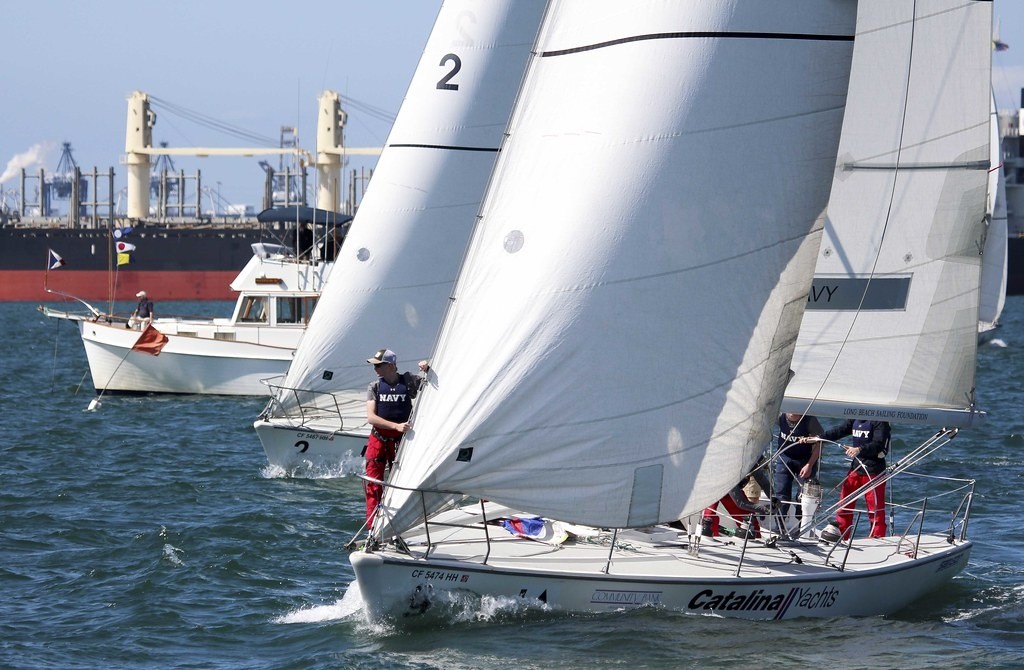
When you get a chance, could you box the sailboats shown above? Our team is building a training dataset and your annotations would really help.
[254,0,549,481]
[342,1,992,630]
[978,89,1008,346]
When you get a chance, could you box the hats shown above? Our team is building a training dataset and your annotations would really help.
[367,349,397,365]
[136,291,146,298]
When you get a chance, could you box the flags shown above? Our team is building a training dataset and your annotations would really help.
[111,227,133,240]
[115,242,136,253]
[49,250,65,271]
[118,254,135,265]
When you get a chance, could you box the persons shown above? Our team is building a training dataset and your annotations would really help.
[824,420,892,538]
[134,290,153,330]
[704,461,781,539]
[320,224,344,261]
[361,348,429,553]
[773,412,825,538]
[293,221,312,260]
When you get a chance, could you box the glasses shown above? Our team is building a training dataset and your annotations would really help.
[374,362,388,368]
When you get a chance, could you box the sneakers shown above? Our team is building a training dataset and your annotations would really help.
[388,536,407,553]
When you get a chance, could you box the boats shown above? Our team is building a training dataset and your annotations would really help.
[37,138,355,397]
[0,90,352,302]
